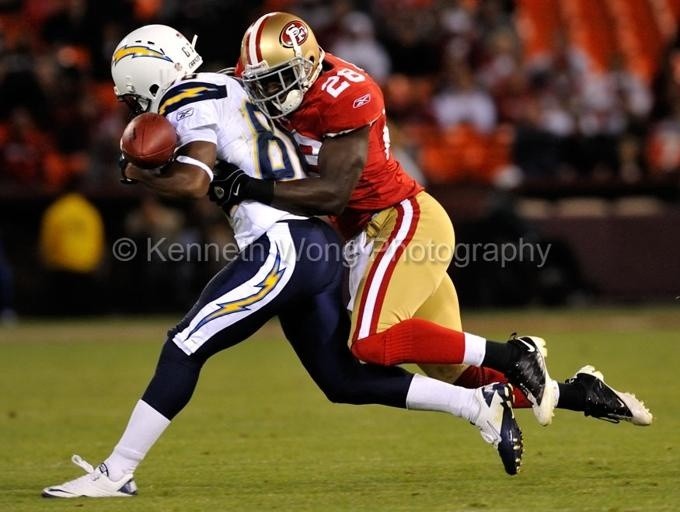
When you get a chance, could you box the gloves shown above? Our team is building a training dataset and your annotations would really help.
[206,158,274,210]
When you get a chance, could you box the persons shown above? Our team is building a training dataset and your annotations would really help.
[203,11,652,426]
[39,22,524,498]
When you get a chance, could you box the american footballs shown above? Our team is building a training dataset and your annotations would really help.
[120,112,177,169]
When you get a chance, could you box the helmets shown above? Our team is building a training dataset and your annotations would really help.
[111,24,203,115]
[242,12,325,119]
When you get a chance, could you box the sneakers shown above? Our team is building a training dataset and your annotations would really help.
[475,382,525,476]
[570,364,653,426]
[42,456,138,498]
[509,336,561,426]
